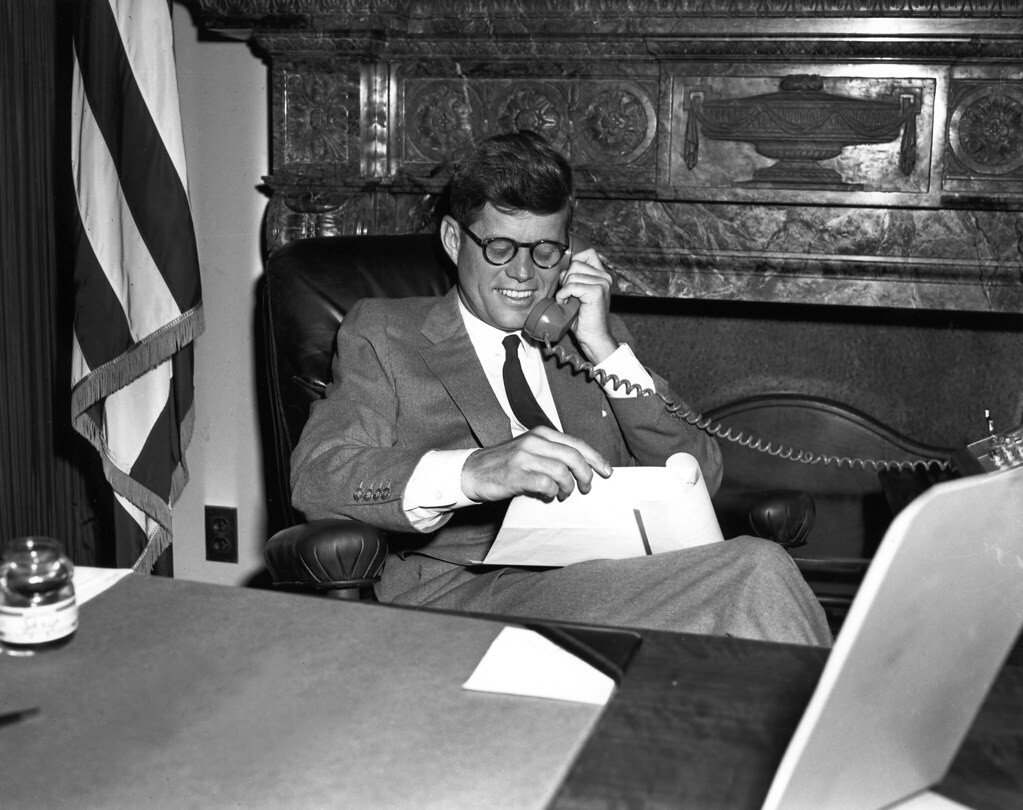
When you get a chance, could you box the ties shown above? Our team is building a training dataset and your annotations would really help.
[502,334,560,432]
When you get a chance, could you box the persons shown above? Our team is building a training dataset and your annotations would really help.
[289,129,834,648]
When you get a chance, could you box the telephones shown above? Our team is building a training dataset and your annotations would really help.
[524,232,590,342]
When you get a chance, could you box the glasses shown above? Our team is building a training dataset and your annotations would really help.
[460,221,570,269]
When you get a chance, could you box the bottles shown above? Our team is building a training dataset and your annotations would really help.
[0,536,79,650]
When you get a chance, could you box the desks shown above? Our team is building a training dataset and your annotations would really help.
[0,567,1023,810]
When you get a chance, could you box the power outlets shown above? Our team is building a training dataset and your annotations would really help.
[205,505,237,563]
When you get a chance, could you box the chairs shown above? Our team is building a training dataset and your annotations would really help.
[263,236,817,603]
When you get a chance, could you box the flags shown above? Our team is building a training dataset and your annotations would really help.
[1,1,205,574]
